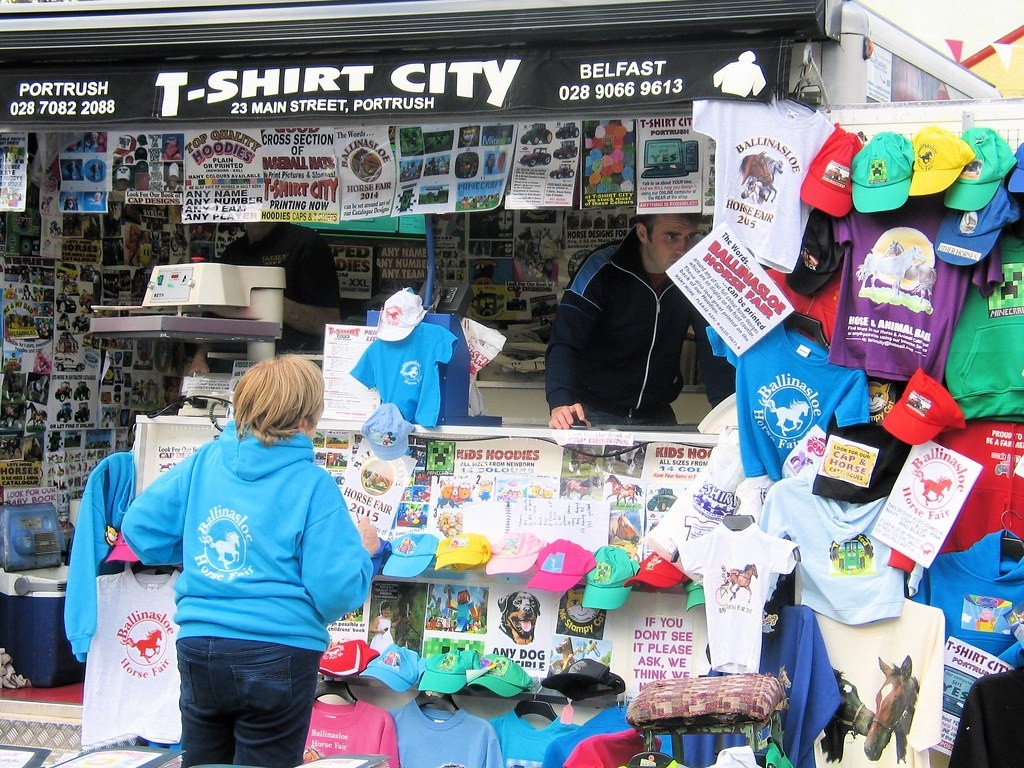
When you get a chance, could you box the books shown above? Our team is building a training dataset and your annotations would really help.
[51,747,187,768]
[0,745,52,768]
[296,753,391,768]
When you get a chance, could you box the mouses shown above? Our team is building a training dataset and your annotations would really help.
[569,417,587,430]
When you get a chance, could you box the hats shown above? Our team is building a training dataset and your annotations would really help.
[786,127,1024,295]
[882,369,967,445]
[376,290,424,341]
[360,403,414,460]
[367,533,704,611]
[320,640,627,702]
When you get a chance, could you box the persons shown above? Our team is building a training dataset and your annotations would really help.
[544,213,736,430]
[122,354,380,768]
[617,216,626,228]
[188,223,340,373]
[450,214,465,241]
[164,163,182,191]
[113,166,134,191]
[473,241,488,255]
[163,137,182,160]
[3,146,11,161]
[539,228,553,256]
[370,601,401,652]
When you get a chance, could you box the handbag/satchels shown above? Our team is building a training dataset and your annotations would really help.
[461,319,506,416]
[627,672,783,733]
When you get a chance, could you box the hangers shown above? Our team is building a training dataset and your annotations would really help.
[415,690,460,712]
[130,559,174,577]
[784,62,816,113]
[782,291,830,354]
[625,728,673,768]
[714,717,785,768]
[670,495,801,563]
[1000,509,1024,563]
[514,680,557,722]
[313,676,357,707]
